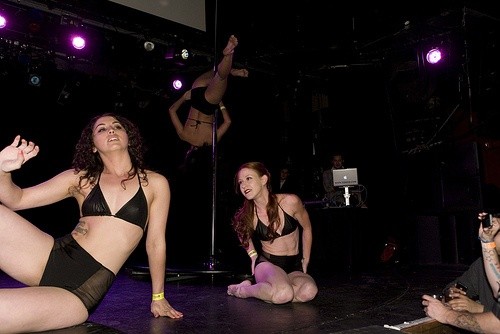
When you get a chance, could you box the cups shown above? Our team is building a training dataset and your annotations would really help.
[449,282,468,299]
[430,294,445,306]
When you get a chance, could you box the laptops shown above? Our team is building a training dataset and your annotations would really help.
[333,168,358,187]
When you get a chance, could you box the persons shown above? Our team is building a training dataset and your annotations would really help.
[226,161,319,304]
[168,35,249,165]
[422,211,500,334]
[274,153,360,202]
[0,112,183,333]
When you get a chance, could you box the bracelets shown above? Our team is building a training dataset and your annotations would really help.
[248,249,257,257]
[481,241,496,248]
[152,291,165,301]
[220,105,226,110]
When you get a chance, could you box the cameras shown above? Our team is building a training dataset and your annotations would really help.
[482,214,492,228]
[455,282,468,292]
[431,295,443,302]
